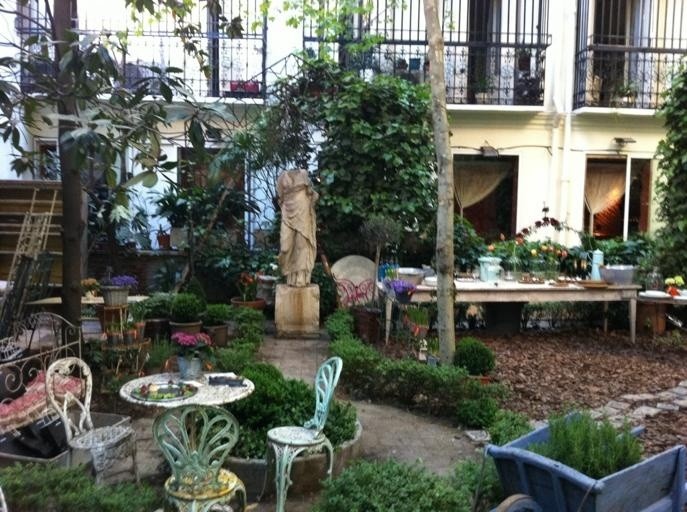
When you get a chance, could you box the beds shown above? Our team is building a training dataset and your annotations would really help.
[0,312,85,446]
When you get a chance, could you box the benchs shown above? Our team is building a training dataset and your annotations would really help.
[225,81,261,98]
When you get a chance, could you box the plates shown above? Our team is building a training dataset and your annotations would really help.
[130,382,199,403]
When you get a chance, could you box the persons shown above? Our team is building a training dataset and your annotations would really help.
[275,167,319,288]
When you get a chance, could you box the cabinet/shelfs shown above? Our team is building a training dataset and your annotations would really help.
[101,306,145,378]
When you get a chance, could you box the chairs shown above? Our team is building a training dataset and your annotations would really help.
[256,356,343,512]
[150,404,248,511]
[320,253,378,312]
[43,357,142,490]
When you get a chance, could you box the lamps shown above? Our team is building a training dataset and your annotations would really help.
[615,134,637,153]
[478,145,499,159]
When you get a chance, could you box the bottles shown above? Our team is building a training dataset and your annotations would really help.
[379,259,399,283]
[591,248,604,283]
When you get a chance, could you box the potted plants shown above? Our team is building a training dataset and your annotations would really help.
[104,290,231,347]
[99,319,145,343]
[214,361,363,500]
[454,336,495,384]
[351,211,403,343]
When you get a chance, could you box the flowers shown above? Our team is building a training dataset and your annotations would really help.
[664,275,685,288]
[99,273,137,289]
[171,330,210,355]
[239,269,257,302]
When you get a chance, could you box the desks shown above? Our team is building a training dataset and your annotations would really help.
[374,277,637,346]
[118,368,256,448]
[637,291,687,335]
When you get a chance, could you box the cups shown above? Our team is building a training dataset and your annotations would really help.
[477,256,502,283]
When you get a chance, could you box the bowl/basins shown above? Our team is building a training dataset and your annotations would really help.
[598,263,640,285]
[397,267,425,286]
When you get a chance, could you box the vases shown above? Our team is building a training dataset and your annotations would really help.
[665,286,680,297]
[103,286,129,305]
[229,297,265,311]
